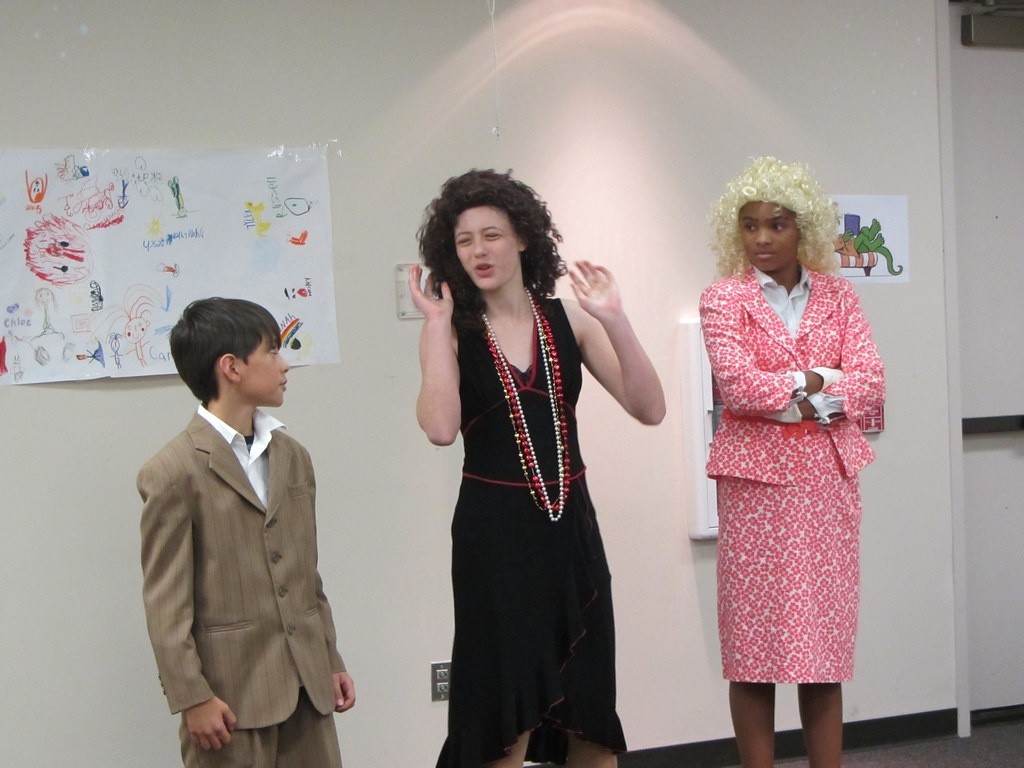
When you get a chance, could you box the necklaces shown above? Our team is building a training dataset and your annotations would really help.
[479,284,573,526]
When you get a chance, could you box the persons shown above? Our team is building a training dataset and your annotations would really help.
[692,152,887,768]
[406,165,667,768]
[139,296,357,768]
